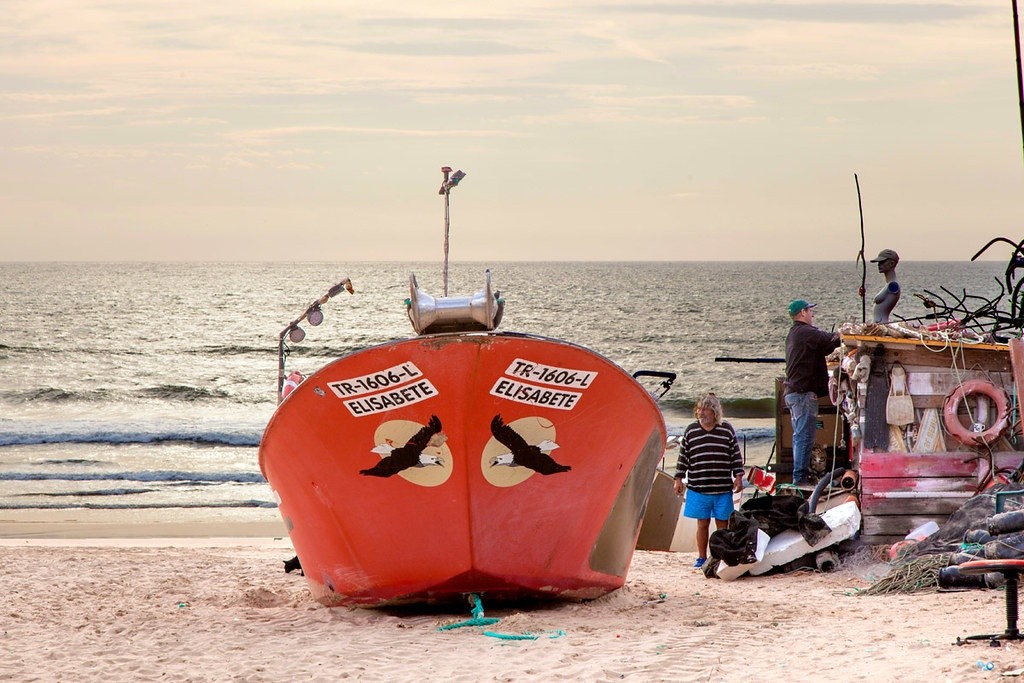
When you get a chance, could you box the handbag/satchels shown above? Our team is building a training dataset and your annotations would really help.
[886,381,914,425]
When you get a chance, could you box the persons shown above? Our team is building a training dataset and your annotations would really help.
[869,249,901,325]
[673,393,745,569]
[782,299,844,486]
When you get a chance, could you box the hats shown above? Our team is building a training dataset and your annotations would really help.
[870,249,900,263]
[788,299,817,316]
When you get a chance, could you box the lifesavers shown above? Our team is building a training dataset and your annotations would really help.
[944,380,1012,447]
[281,370,304,402]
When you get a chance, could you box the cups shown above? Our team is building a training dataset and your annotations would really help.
[973,423,985,432]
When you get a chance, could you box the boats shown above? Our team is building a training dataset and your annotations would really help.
[257,168,678,609]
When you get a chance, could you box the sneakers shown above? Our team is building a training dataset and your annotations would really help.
[694,557,706,570]
